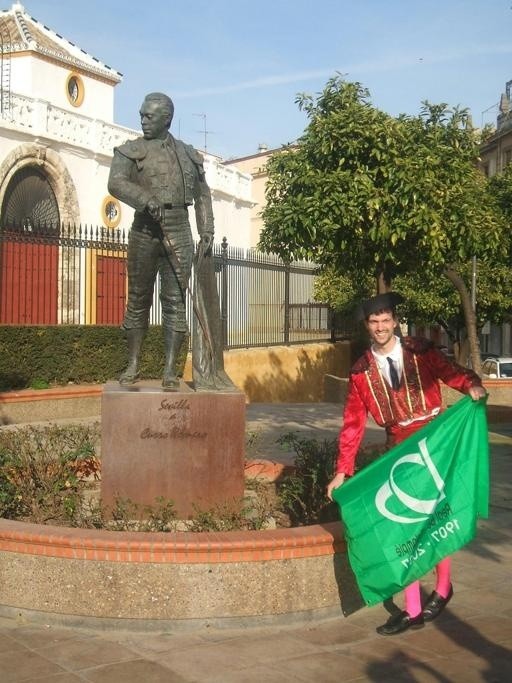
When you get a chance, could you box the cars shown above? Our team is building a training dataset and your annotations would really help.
[482,357,512,380]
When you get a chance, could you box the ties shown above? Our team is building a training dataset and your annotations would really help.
[386,356,401,391]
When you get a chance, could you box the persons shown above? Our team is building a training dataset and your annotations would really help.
[107,92,215,392]
[327,292,487,635]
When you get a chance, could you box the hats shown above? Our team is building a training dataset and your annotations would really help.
[360,291,403,321]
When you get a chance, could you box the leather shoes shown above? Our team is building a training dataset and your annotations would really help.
[421,582,453,622]
[376,610,425,635]
